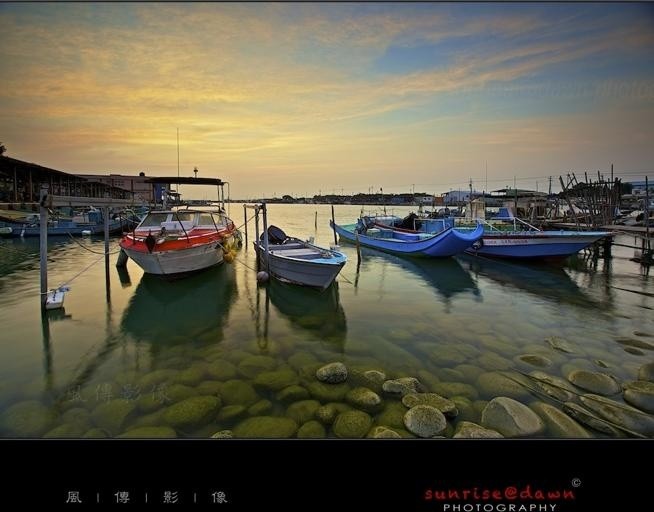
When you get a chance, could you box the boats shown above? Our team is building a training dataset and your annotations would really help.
[0,204,236,273]
[329,215,626,256]
[253,225,348,290]
